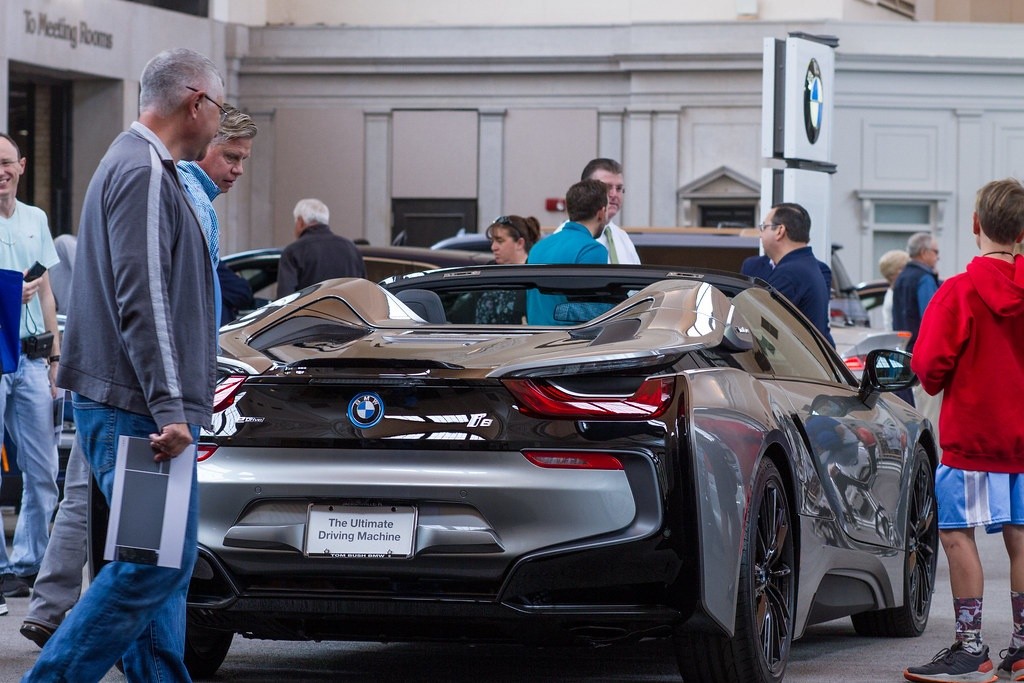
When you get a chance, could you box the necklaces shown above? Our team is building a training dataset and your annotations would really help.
[983,251,1013,256]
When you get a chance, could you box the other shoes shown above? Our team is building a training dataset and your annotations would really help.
[20,621,57,649]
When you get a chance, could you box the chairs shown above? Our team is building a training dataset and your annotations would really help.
[395,289,448,325]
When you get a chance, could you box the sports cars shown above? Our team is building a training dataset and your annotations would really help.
[85,263,942,682]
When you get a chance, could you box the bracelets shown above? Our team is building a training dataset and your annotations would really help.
[48,355,60,363]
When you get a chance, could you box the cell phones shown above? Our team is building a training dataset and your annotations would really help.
[24,261,47,283]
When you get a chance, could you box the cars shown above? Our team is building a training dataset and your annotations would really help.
[220,225,913,412]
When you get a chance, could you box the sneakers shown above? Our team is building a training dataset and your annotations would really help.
[0,573,31,598]
[17,574,37,588]
[903,640,999,683]
[994,646,1024,682]
[0,590,8,615]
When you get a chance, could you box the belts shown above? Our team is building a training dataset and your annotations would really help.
[20,339,28,354]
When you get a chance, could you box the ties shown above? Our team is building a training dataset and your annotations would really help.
[603,226,619,264]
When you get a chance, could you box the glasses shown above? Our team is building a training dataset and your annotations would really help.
[186,85,228,123]
[493,215,523,237]
[925,248,939,255]
[606,183,625,194]
[758,224,781,232]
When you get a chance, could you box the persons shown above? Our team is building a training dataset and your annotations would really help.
[892,233,948,462]
[474,214,541,325]
[524,178,610,325]
[276,199,367,300]
[0,46,257,683]
[555,159,644,297]
[879,250,910,331]
[904,177,1024,683]
[742,254,833,308]
[760,203,828,346]
